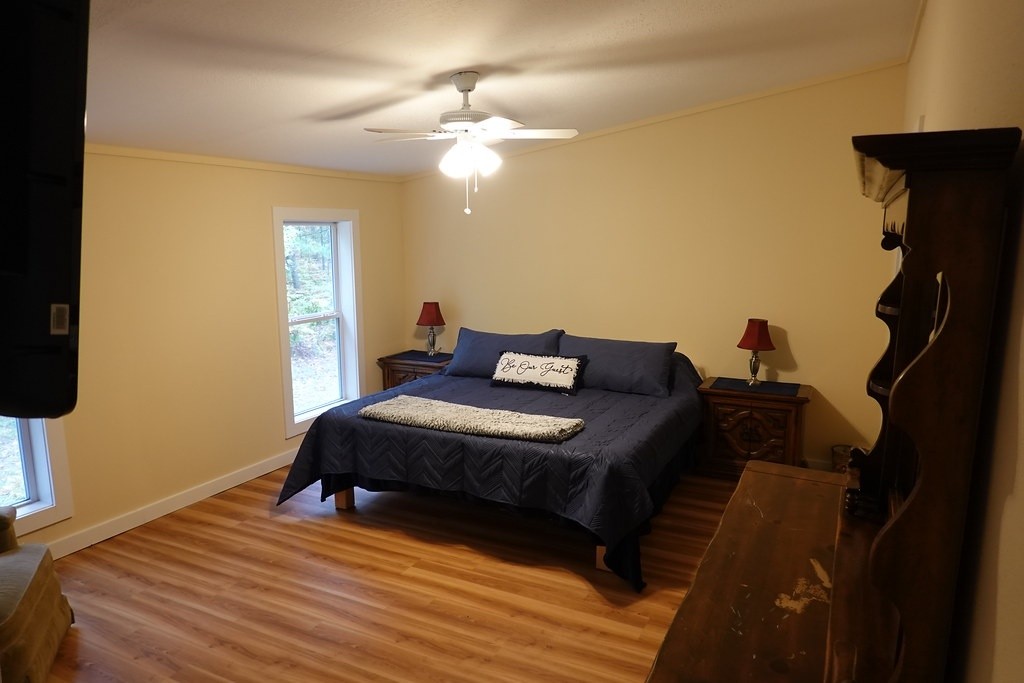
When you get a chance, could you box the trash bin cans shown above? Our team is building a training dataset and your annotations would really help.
[831,444,869,473]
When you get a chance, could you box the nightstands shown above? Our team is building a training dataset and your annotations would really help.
[697,377,812,481]
[377,350,454,391]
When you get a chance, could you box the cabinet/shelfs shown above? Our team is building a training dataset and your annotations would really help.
[642,127,1024,683]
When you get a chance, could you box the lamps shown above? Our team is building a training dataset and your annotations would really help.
[439,134,504,213]
[416,302,446,356]
[736,318,776,387]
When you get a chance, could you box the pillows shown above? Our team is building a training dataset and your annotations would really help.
[558,333,677,397]
[489,351,587,396]
[444,327,567,377]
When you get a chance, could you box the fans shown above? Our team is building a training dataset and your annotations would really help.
[364,71,579,143]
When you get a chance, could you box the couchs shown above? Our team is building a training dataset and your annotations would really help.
[0,506,74,683]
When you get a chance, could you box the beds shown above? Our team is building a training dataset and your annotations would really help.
[275,350,703,592]
